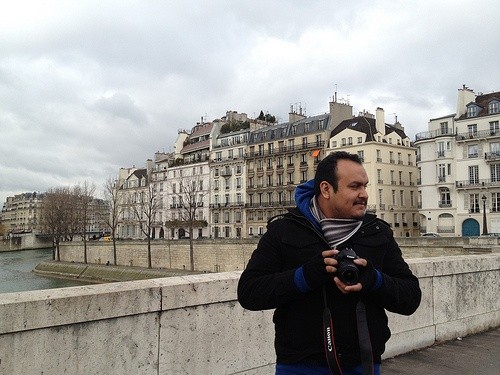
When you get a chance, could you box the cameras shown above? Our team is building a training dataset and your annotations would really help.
[330,247,361,286]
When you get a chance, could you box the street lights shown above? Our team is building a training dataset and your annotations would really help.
[480,194,491,235]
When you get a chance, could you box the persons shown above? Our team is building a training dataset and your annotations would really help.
[237,152,422,375]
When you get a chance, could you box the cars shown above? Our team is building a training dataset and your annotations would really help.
[421,233,440,238]
